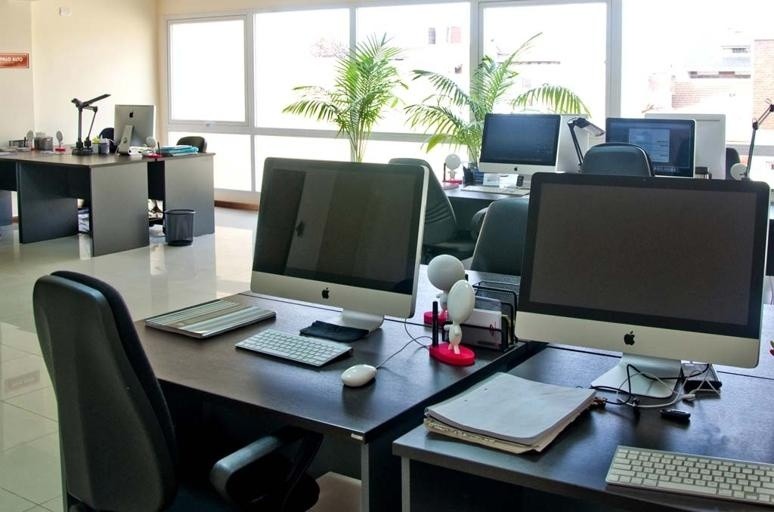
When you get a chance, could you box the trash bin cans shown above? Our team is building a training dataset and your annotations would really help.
[164,209,196,246]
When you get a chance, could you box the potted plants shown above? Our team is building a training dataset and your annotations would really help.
[92,134,101,154]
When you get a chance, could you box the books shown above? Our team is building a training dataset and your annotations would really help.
[424,372,597,455]
[156,145,199,157]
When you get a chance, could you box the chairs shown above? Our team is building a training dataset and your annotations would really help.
[470,197,529,276]
[97,127,116,156]
[578,143,654,178]
[176,136,208,152]
[32,272,320,507]
[388,158,475,262]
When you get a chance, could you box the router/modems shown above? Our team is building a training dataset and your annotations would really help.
[681,363,721,393]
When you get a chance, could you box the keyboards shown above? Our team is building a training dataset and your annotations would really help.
[461,185,531,196]
[606,444,773,507]
[235,328,351,368]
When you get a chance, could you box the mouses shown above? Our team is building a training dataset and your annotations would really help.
[340,363,378,389]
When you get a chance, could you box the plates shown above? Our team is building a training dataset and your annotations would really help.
[129,153,142,156]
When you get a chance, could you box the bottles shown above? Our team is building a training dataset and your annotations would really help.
[694,166,712,179]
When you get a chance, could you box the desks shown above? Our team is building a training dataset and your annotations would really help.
[148,151,217,242]
[439,179,530,225]
[390,346,774,512]
[0,148,156,256]
[134,293,526,509]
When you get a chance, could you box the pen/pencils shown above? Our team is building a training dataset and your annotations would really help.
[432,300,438,347]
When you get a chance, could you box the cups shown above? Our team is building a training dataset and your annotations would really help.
[98,143,110,155]
[128,146,141,154]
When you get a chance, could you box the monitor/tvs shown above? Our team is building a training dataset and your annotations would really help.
[249,156,429,341]
[558,114,589,168]
[604,116,698,176]
[114,104,156,155]
[514,171,770,398]
[476,111,563,188]
[648,113,728,180]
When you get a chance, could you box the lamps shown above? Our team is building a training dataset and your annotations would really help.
[71,94,111,156]
[567,117,605,167]
